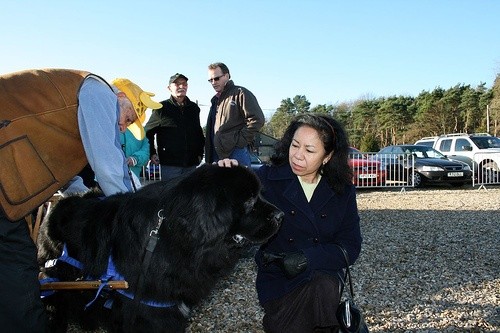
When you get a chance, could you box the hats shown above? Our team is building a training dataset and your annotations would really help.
[113,79,163,141]
[169,73,188,84]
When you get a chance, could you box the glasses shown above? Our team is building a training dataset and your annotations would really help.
[208,74,225,82]
[176,82,187,86]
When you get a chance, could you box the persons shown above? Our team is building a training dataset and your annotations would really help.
[144,73,205,179]
[119,128,150,181]
[0,68,163,333]
[204,62,266,166]
[212,113,363,333]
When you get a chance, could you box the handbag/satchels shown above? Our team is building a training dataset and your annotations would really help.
[327,241,369,333]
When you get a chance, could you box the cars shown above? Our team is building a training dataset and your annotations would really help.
[370,144,473,188]
[348,146,387,189]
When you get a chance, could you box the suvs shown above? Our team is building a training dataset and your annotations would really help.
[414,133,500,183]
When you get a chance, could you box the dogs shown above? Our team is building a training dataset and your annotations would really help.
[36,160,285,333]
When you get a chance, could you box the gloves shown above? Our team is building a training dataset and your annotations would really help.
[260,251,284,267]
[274,250,310,280]
